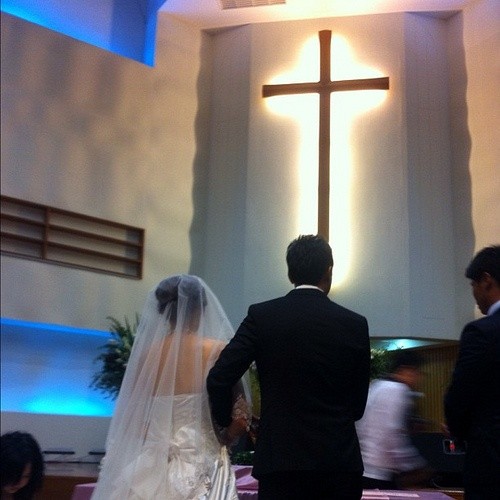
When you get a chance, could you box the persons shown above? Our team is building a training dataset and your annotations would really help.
[443,246,500,500]
[206,234,371,500]
[90,274,251,500]
[0,431,45,500]
[355,352,418,488]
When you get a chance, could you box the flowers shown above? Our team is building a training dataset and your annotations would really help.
[88,313,140,400]
[370,343,397,383]
